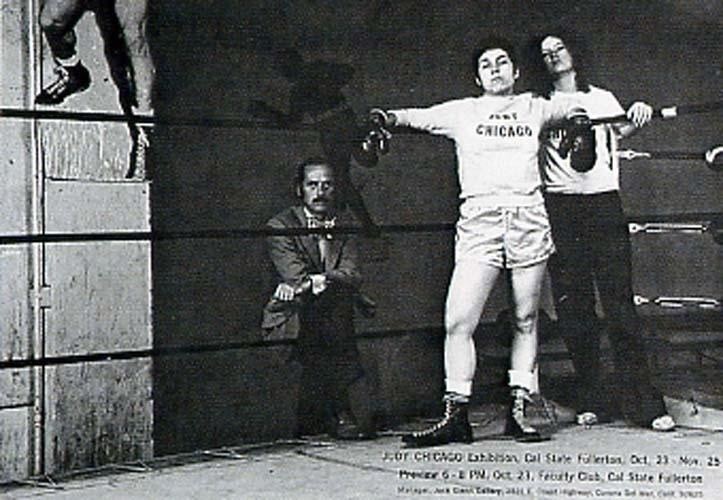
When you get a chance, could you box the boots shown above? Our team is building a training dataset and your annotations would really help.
[402,393,471,442]
[505,386,539,442]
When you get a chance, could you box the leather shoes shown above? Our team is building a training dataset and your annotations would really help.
[329,410,362,439]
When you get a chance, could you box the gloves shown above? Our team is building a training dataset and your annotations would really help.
[559,112,595,172]
[354,108,388,167]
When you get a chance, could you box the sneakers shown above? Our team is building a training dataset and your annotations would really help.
[576,411,597,425]
[35,60,90,104]
[652,415,674,430]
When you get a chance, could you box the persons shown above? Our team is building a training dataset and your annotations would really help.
[523,25,676,433]
[36,0,154,183]
[362,39,597,446]
[265,156,378,440]
